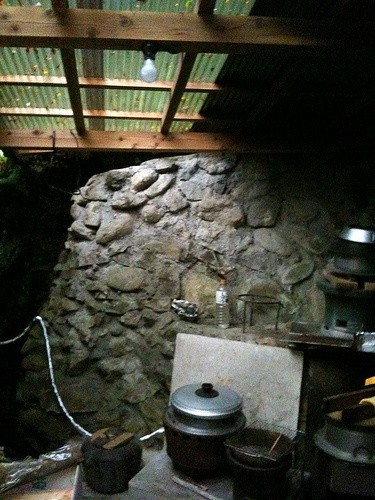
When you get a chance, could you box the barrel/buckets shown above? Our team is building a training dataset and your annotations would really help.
[227,429,297,500]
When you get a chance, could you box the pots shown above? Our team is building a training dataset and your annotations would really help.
[162,382,246,481]
[313,423,375,465]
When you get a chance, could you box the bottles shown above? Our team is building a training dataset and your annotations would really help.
[216,279,230,329]
